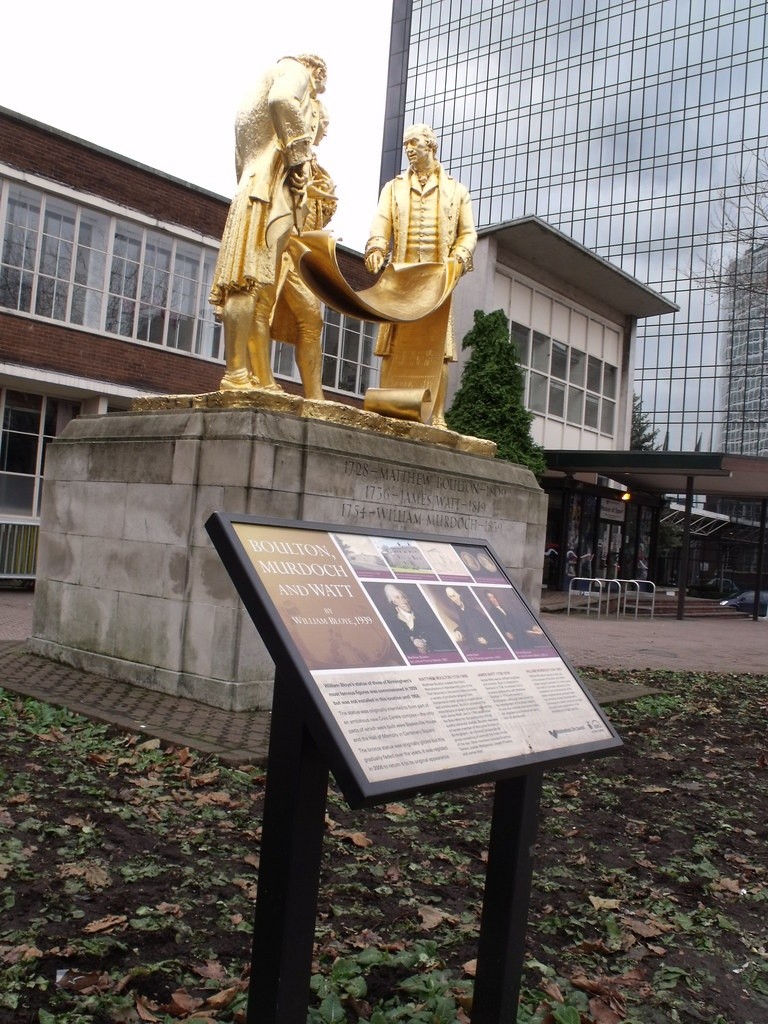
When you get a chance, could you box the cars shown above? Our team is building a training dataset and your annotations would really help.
[706,578,739,594]
[720,590,768,617]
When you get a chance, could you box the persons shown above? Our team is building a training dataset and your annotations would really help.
[364,123,478,428]
[485,590,544,648]
[208,55,339,399]
[383,583,456,652]
[446,587,505,650]
